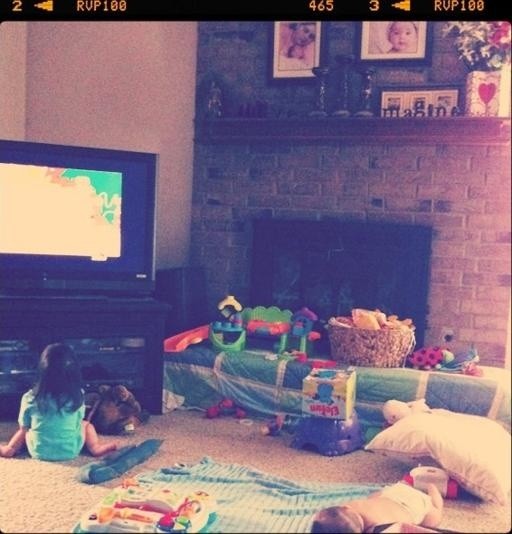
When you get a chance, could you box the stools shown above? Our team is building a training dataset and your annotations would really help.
[290,410,364,457]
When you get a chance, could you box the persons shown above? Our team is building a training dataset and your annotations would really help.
[311,480,445,534]
[386,22,419,53]
[0,341,116,461]
[279,23,316,65]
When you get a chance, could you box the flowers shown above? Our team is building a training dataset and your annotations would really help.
[439,22,512,73]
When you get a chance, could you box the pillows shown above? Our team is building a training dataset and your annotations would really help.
[362,408,512,503]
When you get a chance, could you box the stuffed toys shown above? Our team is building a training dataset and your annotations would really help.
[94,384,141,434]
[407,346,455,370]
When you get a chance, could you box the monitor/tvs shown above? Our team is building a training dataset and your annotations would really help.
[0,139,160,301]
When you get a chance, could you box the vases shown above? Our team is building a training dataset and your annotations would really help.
[462,69,508,118]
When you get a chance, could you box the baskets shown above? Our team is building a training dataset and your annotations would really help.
[327,325,416,368]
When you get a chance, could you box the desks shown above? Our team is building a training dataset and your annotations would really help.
[0,298,170,416]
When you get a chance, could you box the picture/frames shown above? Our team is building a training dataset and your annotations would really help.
[264,21,328,88]
[380,89,459,118]
[355,22,434,66]
